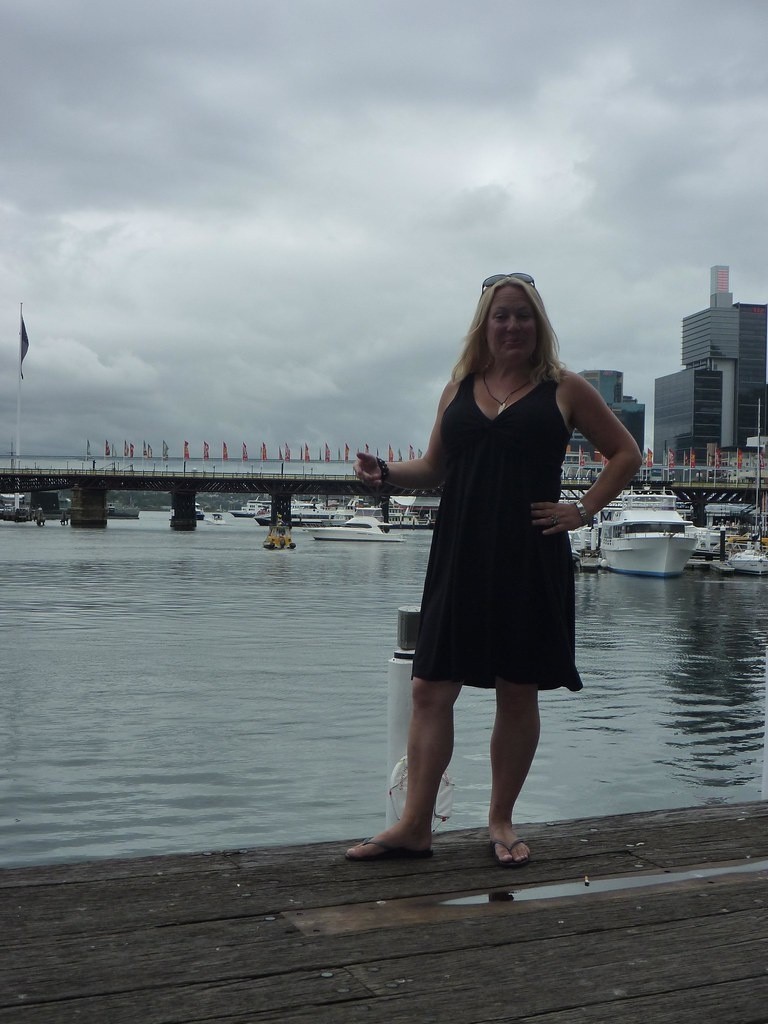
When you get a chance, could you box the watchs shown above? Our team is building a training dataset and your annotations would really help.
[574,500,589,526]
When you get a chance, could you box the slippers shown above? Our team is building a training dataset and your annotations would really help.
[489,824,531,867]
[345,836,435,861]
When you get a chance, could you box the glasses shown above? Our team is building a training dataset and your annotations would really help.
[482,273,536,294]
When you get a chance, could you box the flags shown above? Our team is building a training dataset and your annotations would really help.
[87,437,765,470]
[21,316,29,379]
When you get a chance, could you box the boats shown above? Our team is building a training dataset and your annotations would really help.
[209,514,226,525]
[303,516,408,542]
[728,548,768,575]
[227,500,430,527]
[195,503,205,521]
[568,485,768,578]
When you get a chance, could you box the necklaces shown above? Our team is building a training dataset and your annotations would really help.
[482,366,531,415]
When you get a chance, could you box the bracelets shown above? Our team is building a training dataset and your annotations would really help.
[376,457,390,479]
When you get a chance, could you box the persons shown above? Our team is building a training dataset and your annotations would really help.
[344,277,644,868]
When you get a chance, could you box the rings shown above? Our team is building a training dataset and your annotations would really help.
[551,513,560,526]
